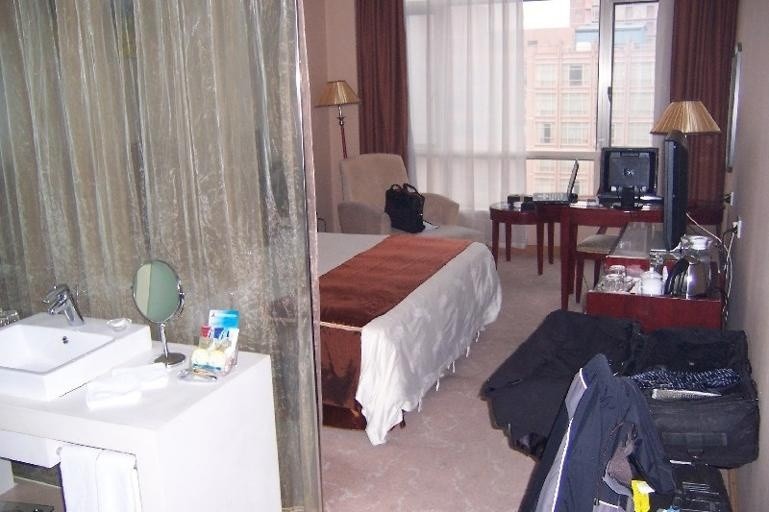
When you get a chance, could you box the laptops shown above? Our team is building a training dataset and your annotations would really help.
[532,160,579,205]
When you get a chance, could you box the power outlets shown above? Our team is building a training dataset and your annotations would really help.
[732,220,741,239]
[728,192,734,206]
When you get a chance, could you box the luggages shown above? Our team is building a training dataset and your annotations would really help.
[480,309,761,469]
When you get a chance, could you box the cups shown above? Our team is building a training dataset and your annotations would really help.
[597,264,628,293]
[636,266,663,296]
[689,235,708,251]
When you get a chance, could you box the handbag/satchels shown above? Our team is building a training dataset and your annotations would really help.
[385,184,426,234]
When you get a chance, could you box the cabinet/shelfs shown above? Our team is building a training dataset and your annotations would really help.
[585,220,724,334]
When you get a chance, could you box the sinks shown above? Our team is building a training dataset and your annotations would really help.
[0,312,152,397]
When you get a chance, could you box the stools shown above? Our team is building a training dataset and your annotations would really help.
[574,223,628,304]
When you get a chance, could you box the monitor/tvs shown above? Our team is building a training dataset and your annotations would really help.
[601,147,659,209]
[665,141,688,249]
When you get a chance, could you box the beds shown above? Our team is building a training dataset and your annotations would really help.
[189,232,491,431]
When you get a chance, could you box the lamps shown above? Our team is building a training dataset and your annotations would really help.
[315,79,363,161]
[650,99,723,138]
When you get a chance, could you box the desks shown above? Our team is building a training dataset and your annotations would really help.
[536,194,577,274]
[489,201,554,276]
[559,200,724,312]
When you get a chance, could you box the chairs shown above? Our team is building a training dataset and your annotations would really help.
[337,153,485,244]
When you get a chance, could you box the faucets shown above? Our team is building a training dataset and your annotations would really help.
[42,284,84,326]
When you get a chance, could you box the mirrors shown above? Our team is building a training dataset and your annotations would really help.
[129,259,185,368]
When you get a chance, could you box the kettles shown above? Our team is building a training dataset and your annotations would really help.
[665,254,709,299]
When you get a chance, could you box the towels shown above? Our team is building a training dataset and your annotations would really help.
[84,362,165,411]
[59,443,142,512]
